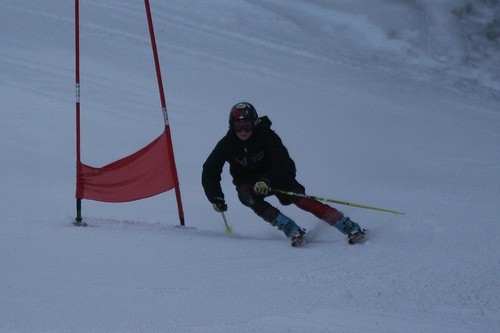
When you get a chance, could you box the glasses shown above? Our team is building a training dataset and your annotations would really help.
[231,121,254,132]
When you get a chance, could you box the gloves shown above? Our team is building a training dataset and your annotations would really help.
[254,178,271,196]
[212,196,227,212]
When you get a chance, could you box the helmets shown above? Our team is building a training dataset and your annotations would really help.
[229,102,258,132]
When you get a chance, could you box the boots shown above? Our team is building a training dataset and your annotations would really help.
[334,214,368,245]
[272,212,306,246]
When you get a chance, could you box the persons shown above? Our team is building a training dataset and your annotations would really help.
[201,102,366,248]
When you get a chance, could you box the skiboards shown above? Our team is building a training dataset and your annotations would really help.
[347,226,370,245]
[291,227,313,248]
